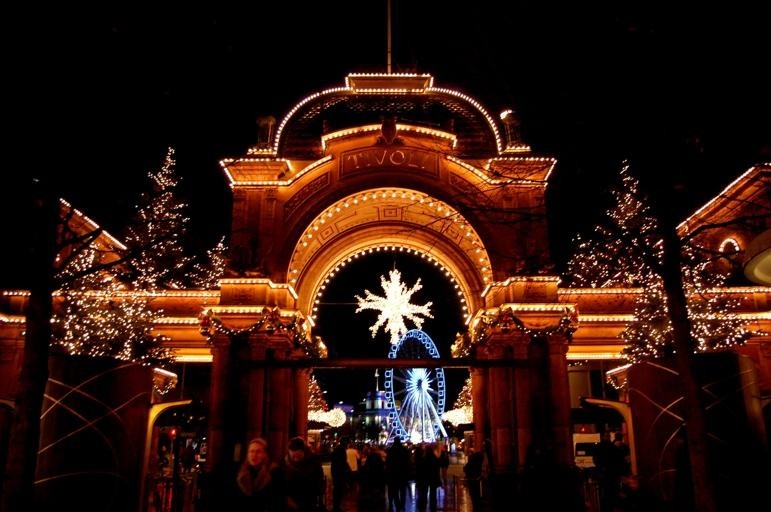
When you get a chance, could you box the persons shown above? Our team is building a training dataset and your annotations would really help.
[227,437,290,511]
[327,432,449,512]
[278,435,324,511]
[462,446,485,511]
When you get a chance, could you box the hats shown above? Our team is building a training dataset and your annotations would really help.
[288,437,304,451]
